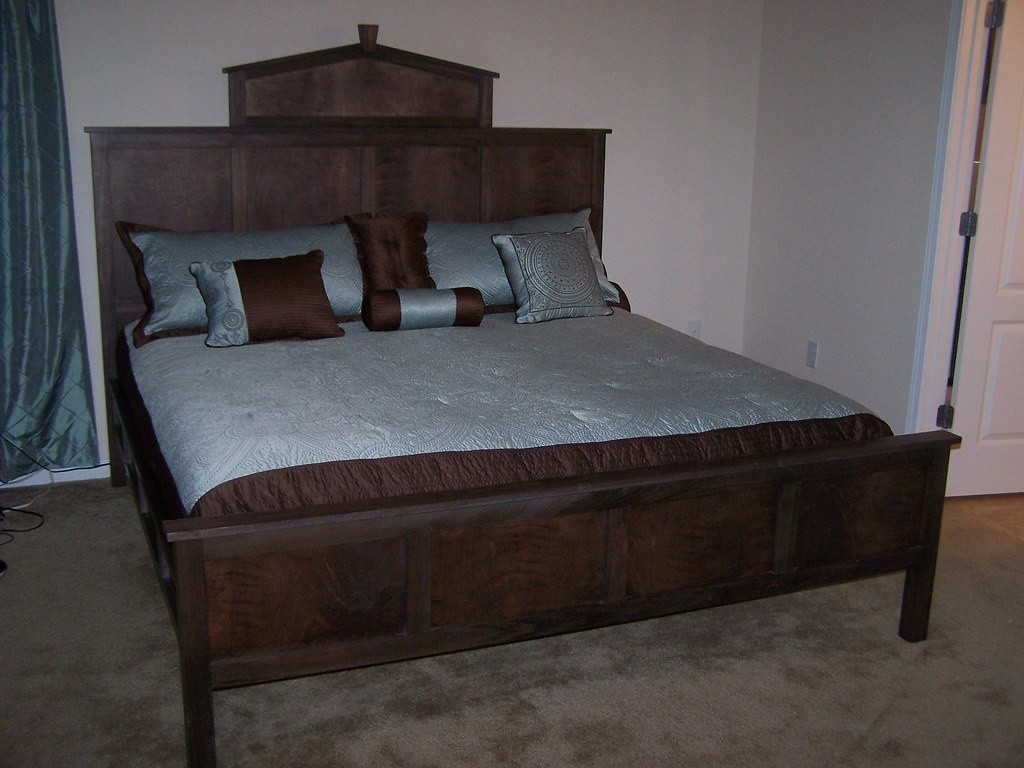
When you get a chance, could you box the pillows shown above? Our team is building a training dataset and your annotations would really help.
[345,210,440,309]
[114,215,375,350]
[360,285,486,331]
[424,207,626,306]
[188,250,347,350]
[491,229,617,324]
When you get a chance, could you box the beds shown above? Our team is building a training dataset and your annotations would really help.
[87,22,963,766]
